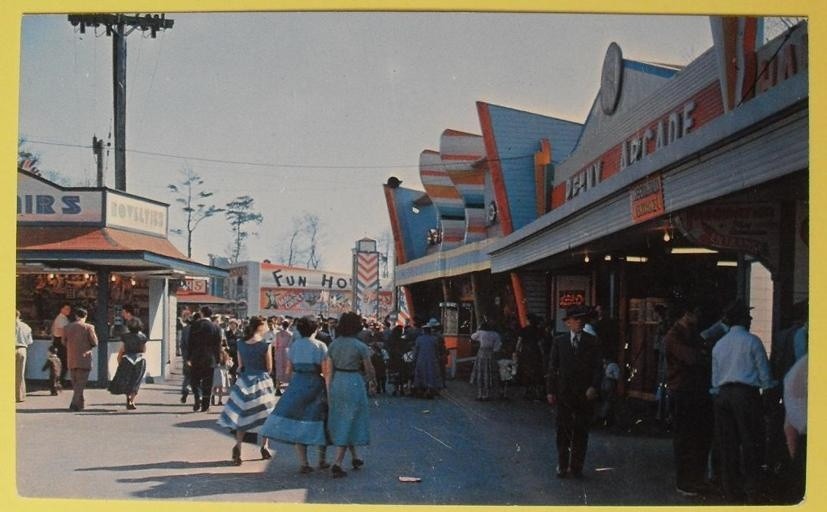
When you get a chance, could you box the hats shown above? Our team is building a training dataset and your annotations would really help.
[561,304,588,321]
[426,318,440,327]
[228,319,239,327]
[727,304,753,318]
[582,323,596,336]
[421,324,432,329]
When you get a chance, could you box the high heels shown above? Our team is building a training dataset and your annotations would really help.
[260,448,271,459]
[232,447,241,464]
[351,458,364,468]
[331,465,347,479]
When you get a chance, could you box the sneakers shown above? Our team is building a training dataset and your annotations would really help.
[194,400,200,410]
[675,482,698,496]
[182,392,190,403]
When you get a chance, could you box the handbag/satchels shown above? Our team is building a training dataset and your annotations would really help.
[498,358,513,381]
[403,351,415,363]
[511,352,519,375]
[605,363,620,379]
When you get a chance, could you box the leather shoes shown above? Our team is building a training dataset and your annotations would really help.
[570,468,584,481]
[275,392,283,396]
[556,464,566,477]
[301,466,314,473]
[318,463,330,469]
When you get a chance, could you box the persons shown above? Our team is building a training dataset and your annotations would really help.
[326,312,377,477]
[50,302,73,390]
[177,306,449,411]
[261,314,332,473]
[61,307,99,410]
[41,345,62,397]
[116,302,136,366]
[469,303,620,480]
[650,294,808,498]
[217,315,276,464]
[15,310,34,403]
[106,317,149,409]
